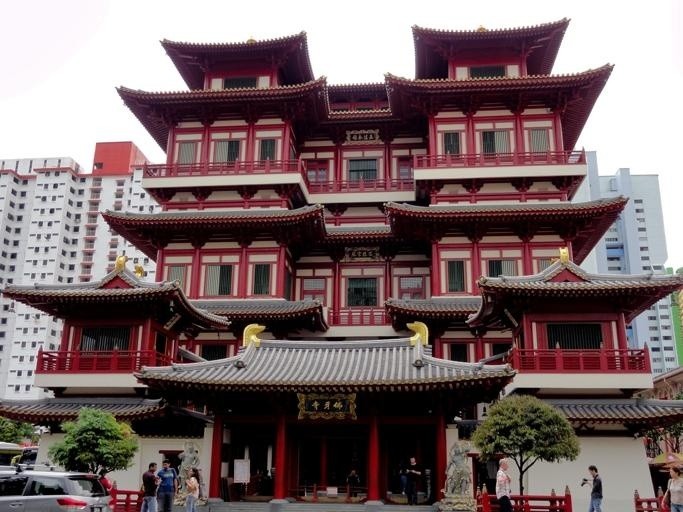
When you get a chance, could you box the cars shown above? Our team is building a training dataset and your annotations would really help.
[0,465,17,482]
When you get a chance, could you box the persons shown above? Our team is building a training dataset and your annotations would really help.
[345,470,360,498]
[156,458,179,512]
[494,458,514,512]
[403,455,423,506]
[140,462,159,511]
[443,442,471,498]
[183,466,200,512]
[581,465,603,512]
[398,462,408,497]
[660,463,682,512]
[94,467,112,495]
[177,445,200,492]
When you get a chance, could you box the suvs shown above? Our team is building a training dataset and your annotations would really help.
[0,463,113,512]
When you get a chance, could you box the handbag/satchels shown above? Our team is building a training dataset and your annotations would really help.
[664,490,670,507]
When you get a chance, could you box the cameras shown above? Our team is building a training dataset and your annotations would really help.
[581,478,588,486]
